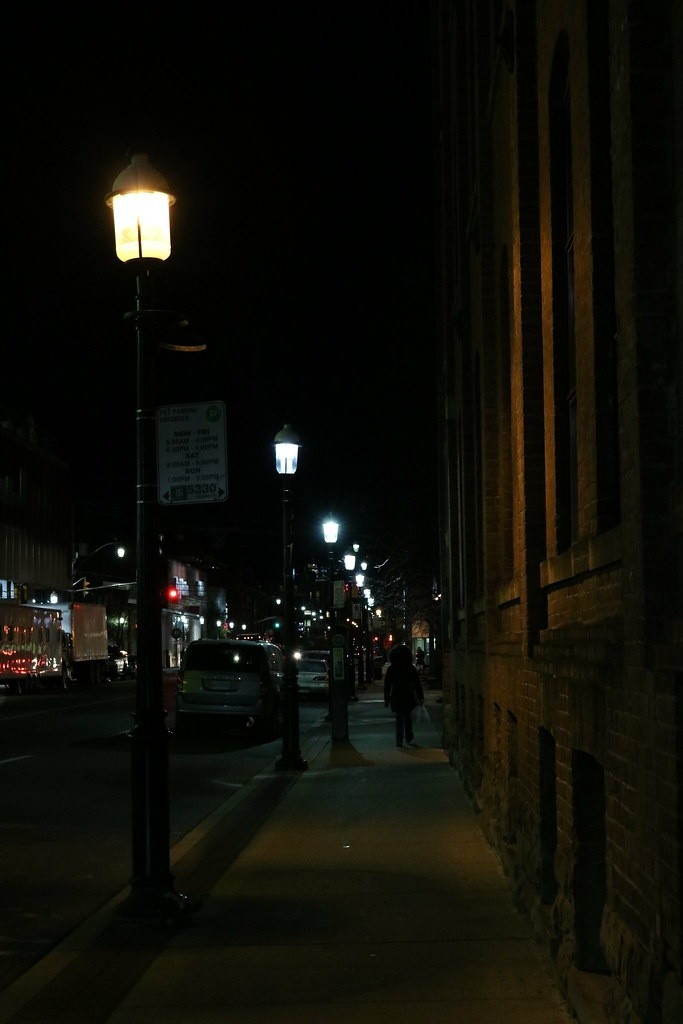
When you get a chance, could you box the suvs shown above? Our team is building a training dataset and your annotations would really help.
[175,637,286,740]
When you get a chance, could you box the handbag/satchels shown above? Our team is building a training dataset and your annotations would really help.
[416,703,431,724]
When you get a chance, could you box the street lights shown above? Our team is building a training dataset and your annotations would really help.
[199,616,205,638]
[106,150,187,937]
[343,548,359,702]
[321,511,342,720]
[216,620,222,638]
[353,572,382,690]
[275,597,281,641]
[229,622,234,638]
[269,423,306,772]
[241,624,246,637]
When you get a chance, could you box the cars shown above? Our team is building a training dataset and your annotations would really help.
[297,660,329,696]
[383,639,392,649]
[105,644,137,679]
[301,649,330,667]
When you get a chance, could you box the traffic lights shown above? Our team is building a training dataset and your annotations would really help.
[274,619,281,629]
[160,587,178,601]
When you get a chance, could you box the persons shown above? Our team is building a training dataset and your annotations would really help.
[384,644,429,747]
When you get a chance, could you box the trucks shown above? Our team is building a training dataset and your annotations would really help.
[0,601,74,693]
